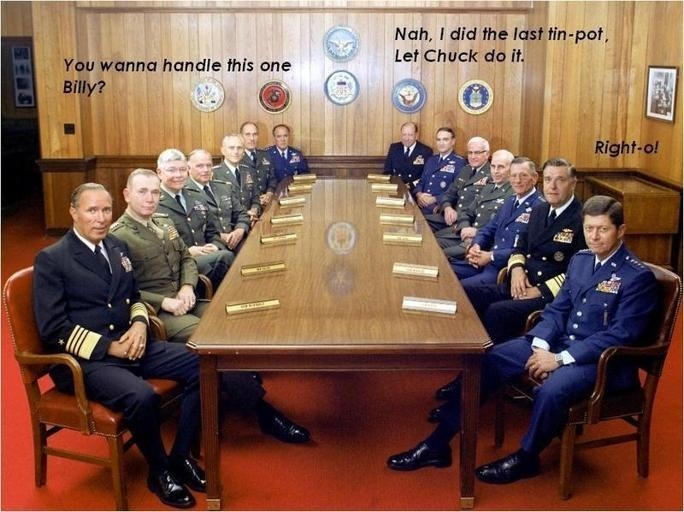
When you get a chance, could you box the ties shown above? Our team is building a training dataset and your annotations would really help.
[403,147,410,159]
[472,168,476,176]
[147,220,164,240]
[492,187,498,192]
[546,209,556,229]
[176,150,285,211]
[594,262,601,272]
[94,245,112,277]
[511,200,519,214]
[440,157,443,163]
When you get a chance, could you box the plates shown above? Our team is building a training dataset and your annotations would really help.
[257,78,293,115]
[391,78,428,113]
[324,220,359,256]
[323,69,361,106]
[321,261,355,299]
[322,24,360,64]
[189,75,225,113]
[457,78,495,115]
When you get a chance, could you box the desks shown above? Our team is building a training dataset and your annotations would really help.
[187,176,492,510]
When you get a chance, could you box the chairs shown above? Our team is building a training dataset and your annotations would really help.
[2,263,184,511]
[432,197,682,499]
[142,273,213,315]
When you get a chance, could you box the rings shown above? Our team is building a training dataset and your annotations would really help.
[138,344,145,348]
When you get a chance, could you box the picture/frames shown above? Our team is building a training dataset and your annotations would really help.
[644,65,680,125]
[9,43,36,109]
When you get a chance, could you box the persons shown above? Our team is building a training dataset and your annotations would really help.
[383,123,433,188]
[107,122,311,445]
[413,128,582,421]
[387,195,655,484]
[31,183,209,509]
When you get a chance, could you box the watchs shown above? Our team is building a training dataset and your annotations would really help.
[553,352,563,367]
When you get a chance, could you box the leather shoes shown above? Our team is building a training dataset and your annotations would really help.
[264,412,309,444]
[435,378,462,401]
[430,402,448,422]
[167,455,206,492]
[147,470,194,507]
[474,448,541,483]
[387,440,452,471]
